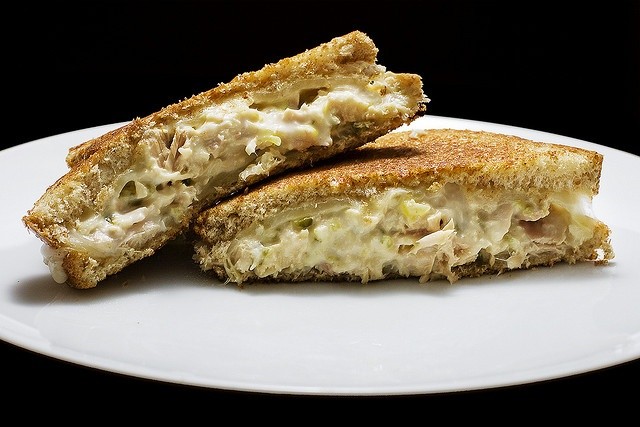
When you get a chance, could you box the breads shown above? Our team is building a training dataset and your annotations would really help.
[22,29,431,292]
[186,128,615,283]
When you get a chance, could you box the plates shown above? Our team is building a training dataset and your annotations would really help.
[1,115,640,396]
